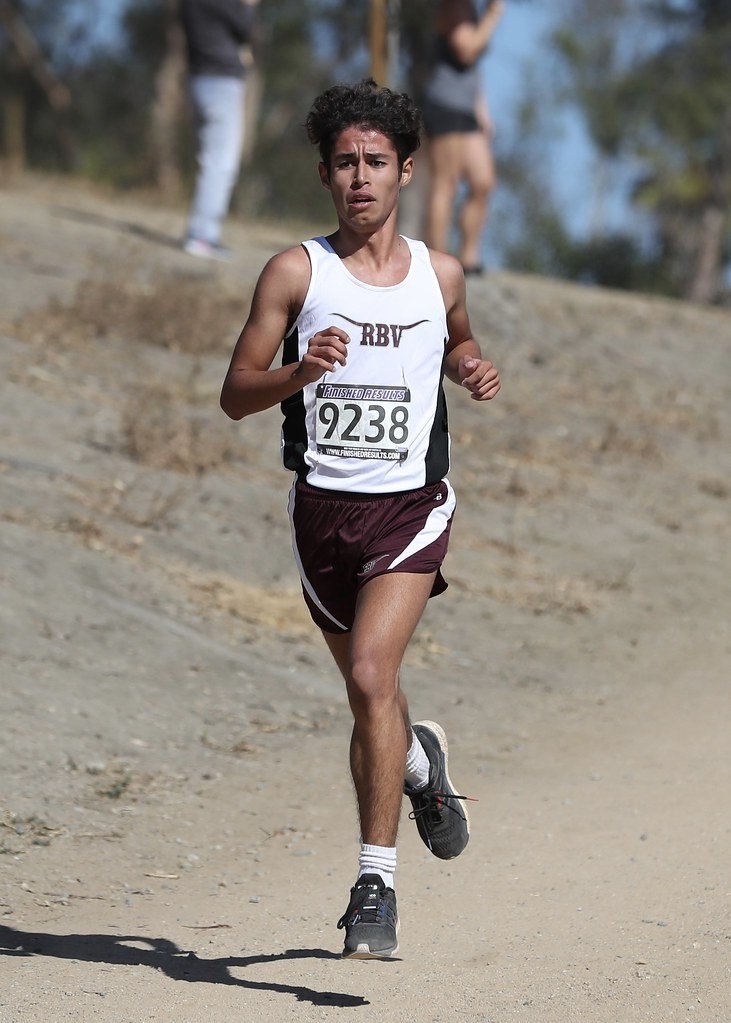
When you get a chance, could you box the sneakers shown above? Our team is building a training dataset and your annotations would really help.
[341,873,399,960]
[403,719,470,862]
[189,234,247,262]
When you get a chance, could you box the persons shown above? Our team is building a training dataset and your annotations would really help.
[399,0,507,277]
[175,0,261,260]
[217,78,503,958]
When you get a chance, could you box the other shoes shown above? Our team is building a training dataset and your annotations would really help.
[459,264,497,288]
